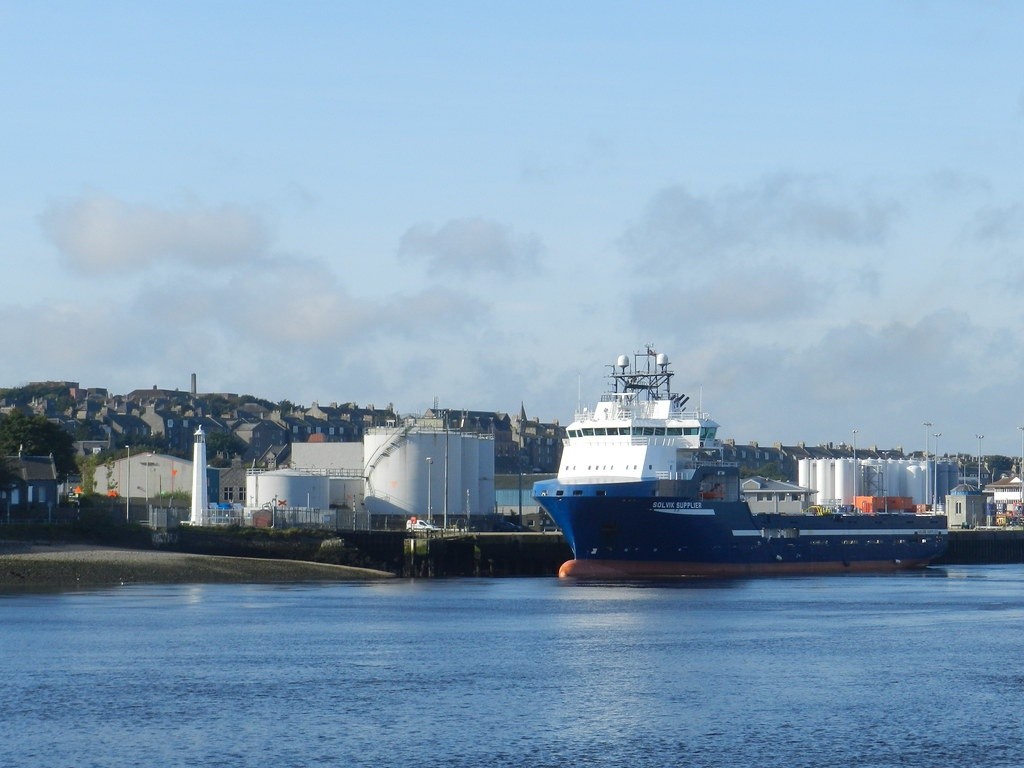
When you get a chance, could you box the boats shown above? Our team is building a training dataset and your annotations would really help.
[530,342,951,581]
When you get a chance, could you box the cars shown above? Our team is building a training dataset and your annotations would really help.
[501,522,523,532]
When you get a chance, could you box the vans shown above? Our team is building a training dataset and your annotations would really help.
[406,520,434,532]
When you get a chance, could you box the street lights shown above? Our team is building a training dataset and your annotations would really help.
[932,433,941,516]
[1017,427,1024,498]
[976,435,985,490]
[852,430,858,512]
[125,445,130,522]
[882,452,889,513]
[426,457,431,524]
[923,423,932,504]
